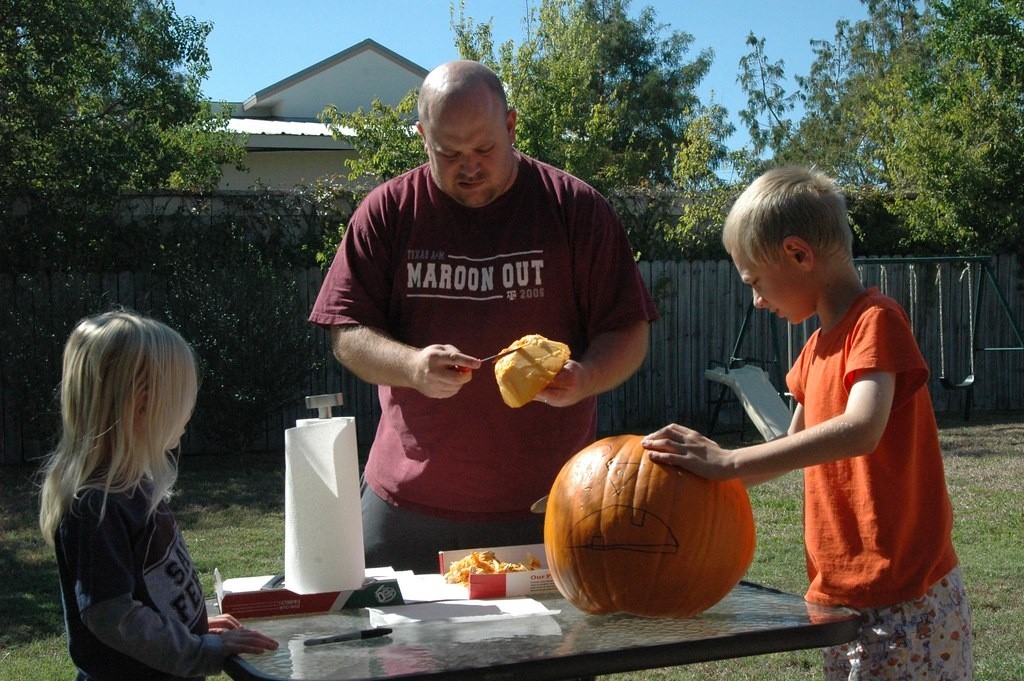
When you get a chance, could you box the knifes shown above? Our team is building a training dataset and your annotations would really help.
[448,345,524,373]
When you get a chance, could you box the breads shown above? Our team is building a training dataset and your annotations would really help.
[490,333,571,406]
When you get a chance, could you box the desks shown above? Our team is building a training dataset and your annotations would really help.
[202,567,865,681]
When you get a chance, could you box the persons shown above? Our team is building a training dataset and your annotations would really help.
[309,60,663,576]
[38,309,283,679]
[639,165,977,679]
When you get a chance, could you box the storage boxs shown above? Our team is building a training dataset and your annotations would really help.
[438,544,559,602]
[212,567,406,619]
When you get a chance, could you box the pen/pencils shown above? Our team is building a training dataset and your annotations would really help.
[302,627,393,647]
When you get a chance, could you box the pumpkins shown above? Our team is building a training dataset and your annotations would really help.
[541,426,756,619]
